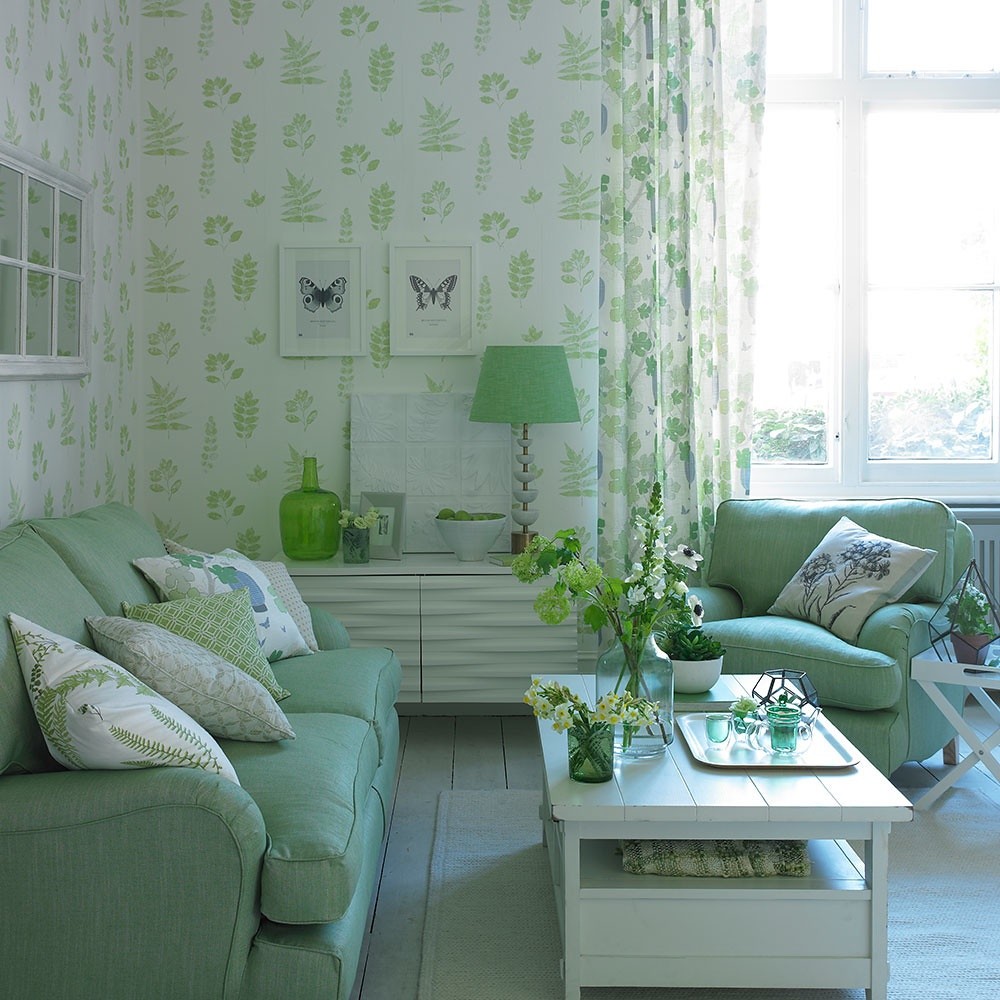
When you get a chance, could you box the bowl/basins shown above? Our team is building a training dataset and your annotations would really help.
[434,512,507,562]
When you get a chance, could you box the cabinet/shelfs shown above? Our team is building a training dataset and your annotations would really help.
[268,552,577,702]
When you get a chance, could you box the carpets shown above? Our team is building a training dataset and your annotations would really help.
[417,786,1000,1000]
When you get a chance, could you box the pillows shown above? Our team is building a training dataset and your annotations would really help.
[8,539,319,786]
[767,516,939,646]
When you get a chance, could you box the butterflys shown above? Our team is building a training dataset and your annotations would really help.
[299,276,347,313]
[410,275,457,312]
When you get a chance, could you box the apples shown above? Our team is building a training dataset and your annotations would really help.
[437,508,501,521]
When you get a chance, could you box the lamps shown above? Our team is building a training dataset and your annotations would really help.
[469,345,582,554]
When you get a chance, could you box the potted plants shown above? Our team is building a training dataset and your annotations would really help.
[945,576,999,665]
[656,630,727,693]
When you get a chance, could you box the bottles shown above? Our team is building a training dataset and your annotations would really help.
[278,457,341,561]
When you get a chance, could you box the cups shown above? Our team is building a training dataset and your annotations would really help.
[705,714,731,743]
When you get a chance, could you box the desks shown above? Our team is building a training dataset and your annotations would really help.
[532,674,914,1000]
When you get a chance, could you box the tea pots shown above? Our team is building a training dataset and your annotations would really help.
[744,694,823,757]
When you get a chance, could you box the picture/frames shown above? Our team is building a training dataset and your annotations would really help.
[360,491,407,560]
[278,242,477,356]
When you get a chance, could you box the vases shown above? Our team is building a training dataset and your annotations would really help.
[279,457,370,563]
[567,633,674,783]
[567,724,614,784]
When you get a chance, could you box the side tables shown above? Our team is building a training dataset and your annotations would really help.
[911,639,1000,810]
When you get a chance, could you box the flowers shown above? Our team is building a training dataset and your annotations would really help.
[338,507,379,530]
[523,677,660,778]
[512,482,705,753]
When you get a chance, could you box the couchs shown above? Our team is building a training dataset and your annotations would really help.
[647,498,975,779]
[0,503,404,1000]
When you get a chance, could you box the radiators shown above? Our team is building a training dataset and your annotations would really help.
[967,525,1000,651]
